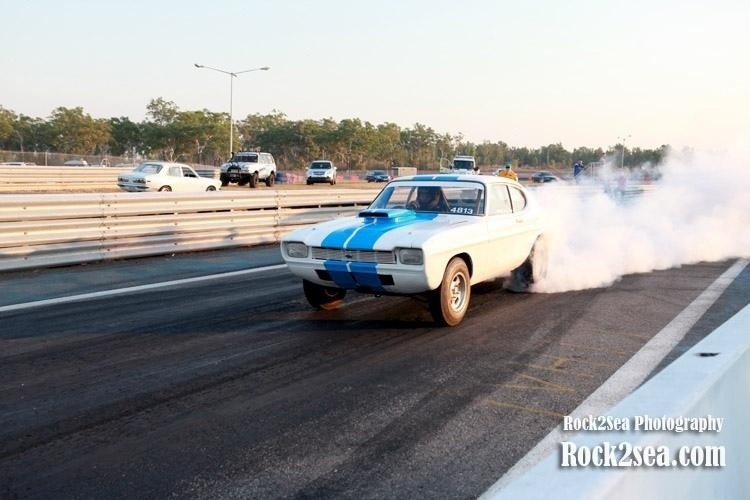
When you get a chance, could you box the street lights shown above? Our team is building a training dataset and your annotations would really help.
[195,62,271,162]
[616,134,632,171]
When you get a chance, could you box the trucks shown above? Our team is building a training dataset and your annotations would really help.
[440,155,481,176]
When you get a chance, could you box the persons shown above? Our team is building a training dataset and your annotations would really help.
[408,187,450,213]
[613,171,626,199]
[575,160,585,188]
[497,164,518,181]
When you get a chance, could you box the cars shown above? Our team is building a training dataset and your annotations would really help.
[1,159,140,168]
[117,162,222,192]
[492,168,506,177]
[531,171,559,184]
[279,174,552,327]
[366,171,390,182]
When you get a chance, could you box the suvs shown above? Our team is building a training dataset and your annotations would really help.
[220,151,278,188]
[304,160,338,186]
